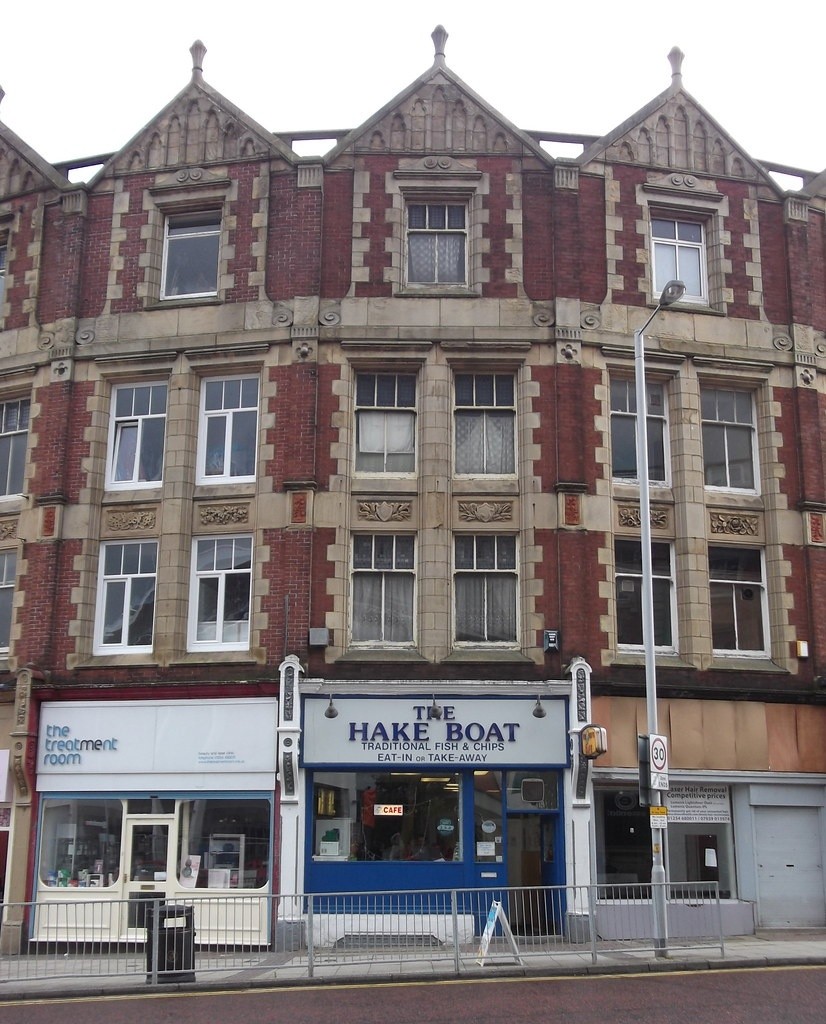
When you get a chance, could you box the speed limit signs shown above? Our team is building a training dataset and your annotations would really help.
[651,738,667,769]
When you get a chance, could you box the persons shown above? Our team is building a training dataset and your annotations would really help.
[389,833,404,861]
[411,836,431,861]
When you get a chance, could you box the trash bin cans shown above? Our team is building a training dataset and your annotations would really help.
[145,904,198,983]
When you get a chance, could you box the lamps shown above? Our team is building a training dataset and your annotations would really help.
[325,692,339,719]
[532,693,547,718]
[429,694,443,719]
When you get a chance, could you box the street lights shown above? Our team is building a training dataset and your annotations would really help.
[630,279,687,952]
